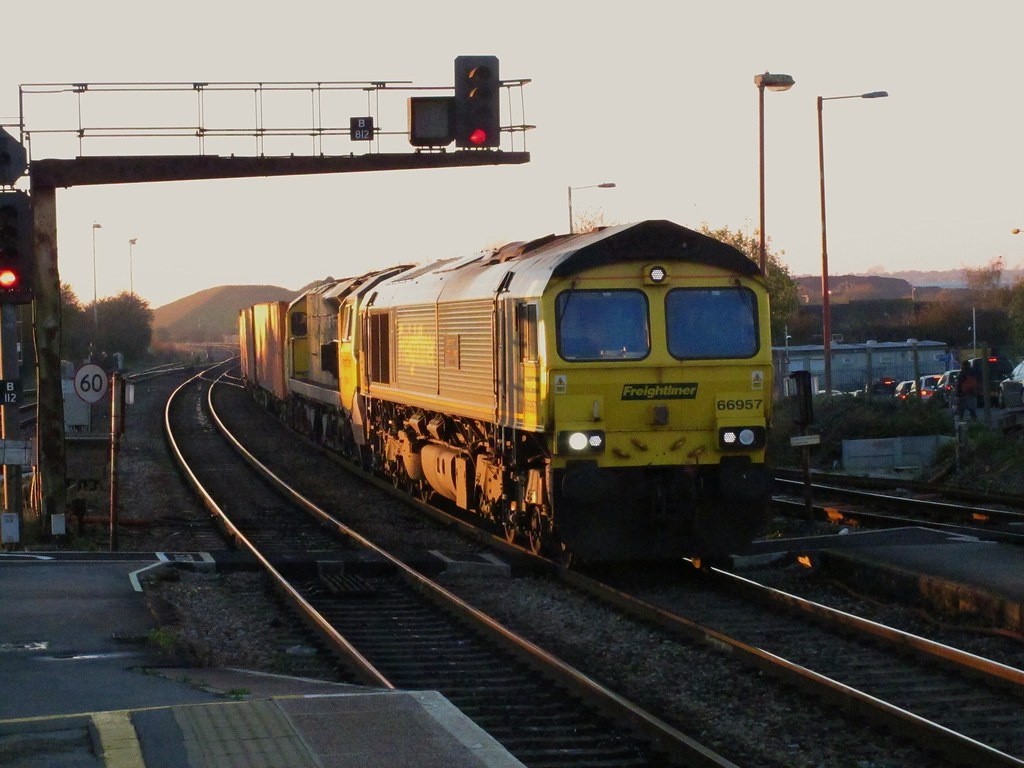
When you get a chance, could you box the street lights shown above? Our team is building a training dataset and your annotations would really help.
[127,237,137,295]
[817,91,890,395]
[92,220,101,330]
[754,72,797,278]
[568,182,616,235]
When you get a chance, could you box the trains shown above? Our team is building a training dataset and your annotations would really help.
[238,221,775,572]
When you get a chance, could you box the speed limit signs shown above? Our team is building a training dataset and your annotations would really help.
[75,364,108,403]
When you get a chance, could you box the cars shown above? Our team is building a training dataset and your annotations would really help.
[817,353,1024,408]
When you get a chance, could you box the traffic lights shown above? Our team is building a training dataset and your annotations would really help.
[0,191,35,305]
[453,55,501,149]
